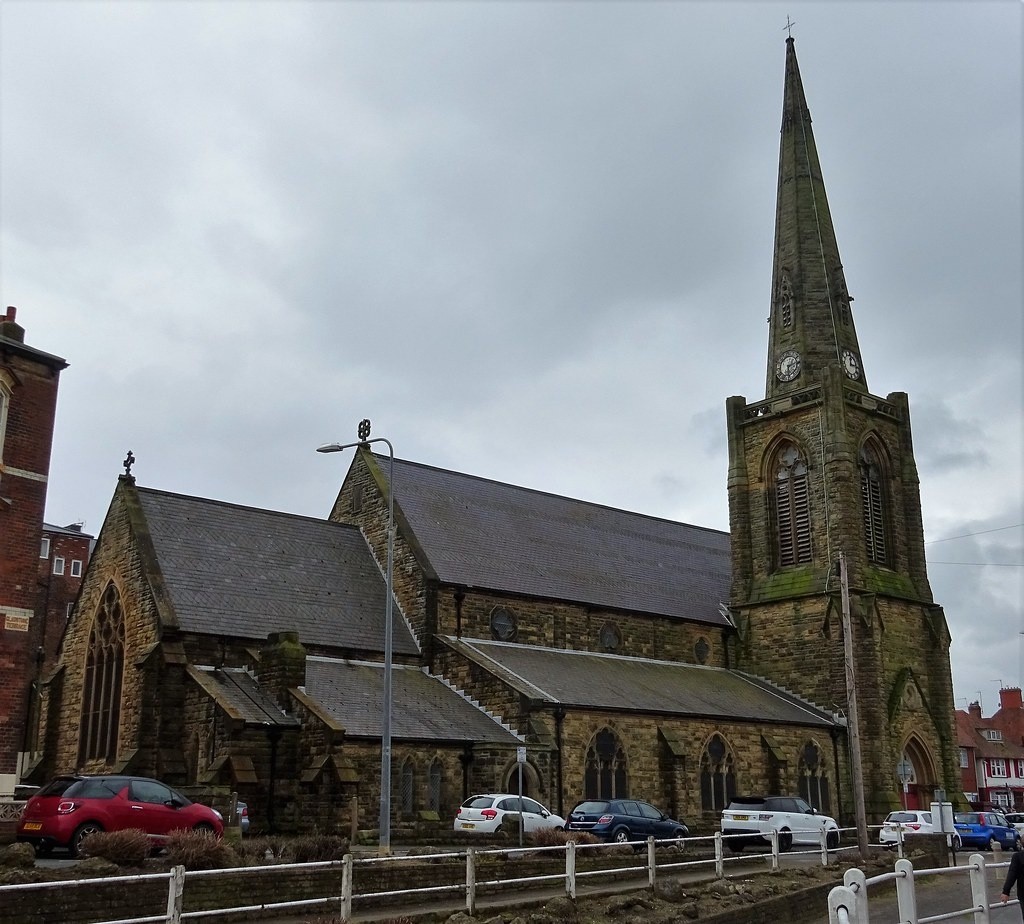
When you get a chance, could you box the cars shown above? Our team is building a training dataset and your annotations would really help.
[15,774,225,858]
[879,810,963,850]
[564,800,691,852]
[237,801,250,835]
[453,793,565,837]
[950,811,1024,850]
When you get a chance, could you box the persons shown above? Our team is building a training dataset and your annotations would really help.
[1001,838,1024,924]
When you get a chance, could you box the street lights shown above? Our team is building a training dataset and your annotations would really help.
[317,438,393,858]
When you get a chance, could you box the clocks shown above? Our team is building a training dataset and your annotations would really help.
[841,349,860,380]
[775,350,801,382]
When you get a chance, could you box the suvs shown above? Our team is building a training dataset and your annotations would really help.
[720,794,843,855]
